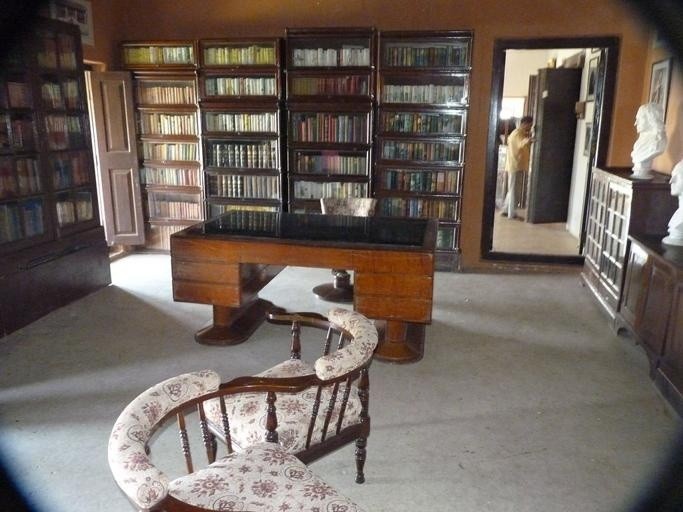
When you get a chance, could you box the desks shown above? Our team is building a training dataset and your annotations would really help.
[170,209,440,365]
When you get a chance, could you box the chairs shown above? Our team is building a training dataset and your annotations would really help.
[312,197,380,304]
[198,308,378,487]
[106,370,369,512]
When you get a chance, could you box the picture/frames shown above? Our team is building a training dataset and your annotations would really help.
[645,59,671,126]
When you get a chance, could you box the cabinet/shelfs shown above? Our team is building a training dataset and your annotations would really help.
[195,36,282,223]
[1,18,49,245]
[578,164,680,336]
[281,27,375,220]
[25,22,101,231]
[376,31,473,274]
[612,237,682,418]
[122,40,203,255]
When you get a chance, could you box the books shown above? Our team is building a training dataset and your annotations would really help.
[124,45,205,250]
[290,47,371,214]
[1,31,92,245]
[379,46,470,250]
[203,46,282,218]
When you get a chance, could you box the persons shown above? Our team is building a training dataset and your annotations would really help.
[498,115,539,222]
[630,101,665,166]
[667,160,683,231]
